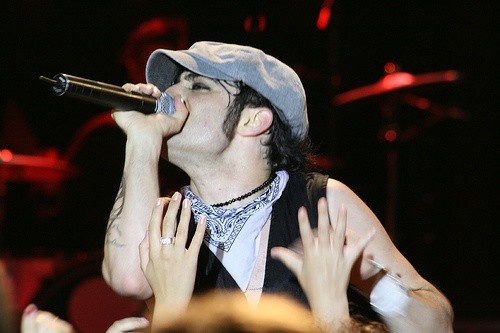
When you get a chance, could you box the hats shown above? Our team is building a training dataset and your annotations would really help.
[144,39,310,143]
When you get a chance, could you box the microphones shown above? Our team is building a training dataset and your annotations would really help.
[39,72,175,116]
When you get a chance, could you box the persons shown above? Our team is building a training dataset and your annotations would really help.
[18,303,149,333]
[100,40,454,333]
[138,192,375,333]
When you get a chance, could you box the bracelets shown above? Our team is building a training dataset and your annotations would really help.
[365,273,410,318]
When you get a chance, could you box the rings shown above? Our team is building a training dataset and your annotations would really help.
[159,237,175,245]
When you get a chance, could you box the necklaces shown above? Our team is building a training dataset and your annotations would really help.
[209,174,277,207]
